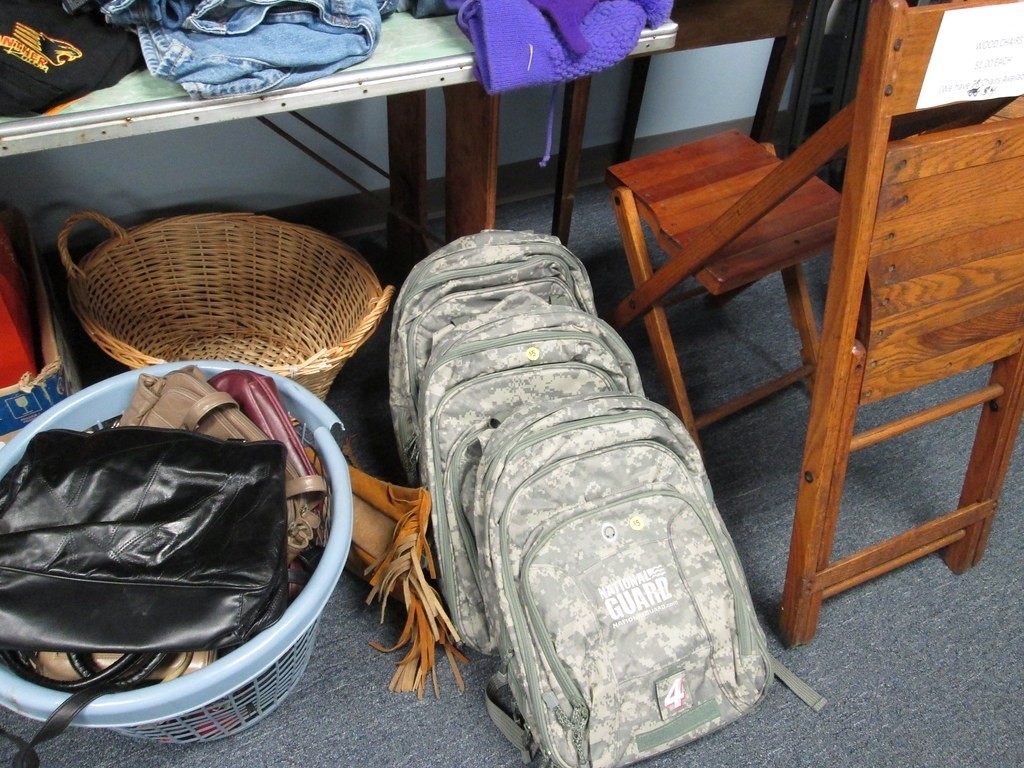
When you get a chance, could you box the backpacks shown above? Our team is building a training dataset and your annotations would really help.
[387,228,828,768]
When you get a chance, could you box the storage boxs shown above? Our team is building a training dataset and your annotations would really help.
[0,201,84,436]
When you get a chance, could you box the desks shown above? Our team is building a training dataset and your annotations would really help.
[0,13,680,348]
[552,0,808,248]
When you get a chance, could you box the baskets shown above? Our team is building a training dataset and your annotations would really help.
[0,362,355,744]
[58,210,393,428]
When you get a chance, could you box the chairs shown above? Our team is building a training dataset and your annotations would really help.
[604,0,1024,651]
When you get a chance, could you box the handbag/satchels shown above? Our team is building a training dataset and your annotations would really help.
[0,366,329,742]
